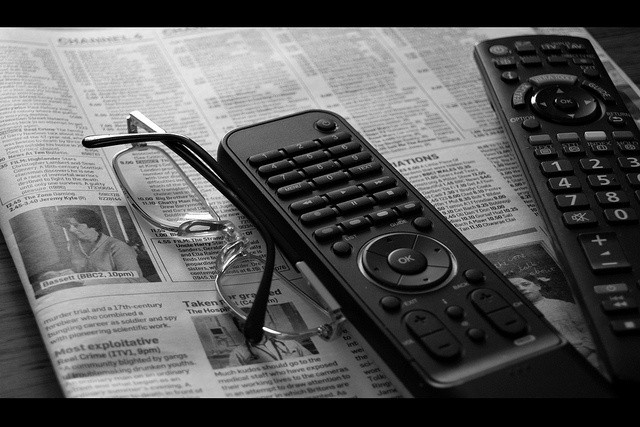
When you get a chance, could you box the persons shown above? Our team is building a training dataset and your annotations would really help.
[227,306,315,366]
[504,272,601,369]
[37,207,144,284]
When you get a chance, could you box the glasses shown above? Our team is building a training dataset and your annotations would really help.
[82,110,347,345]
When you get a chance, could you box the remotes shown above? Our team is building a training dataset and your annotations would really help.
[218,109,627,397]
[472,36,639,397]
